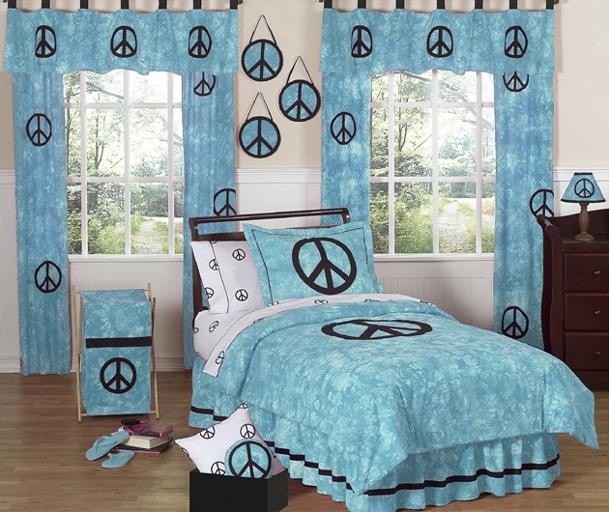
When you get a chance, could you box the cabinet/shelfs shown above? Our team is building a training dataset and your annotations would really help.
[536,208,608,393]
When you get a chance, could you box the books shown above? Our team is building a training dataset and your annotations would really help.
[112,444,169,455]
[117,420,178,436]
[109,430,171,449]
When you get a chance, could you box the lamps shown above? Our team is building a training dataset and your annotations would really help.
[560,169,607,241]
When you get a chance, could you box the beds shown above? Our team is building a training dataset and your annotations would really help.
[186,205,573,512]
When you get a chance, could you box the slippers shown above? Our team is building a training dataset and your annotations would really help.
[102,449,135,469]
[85,430,128,461]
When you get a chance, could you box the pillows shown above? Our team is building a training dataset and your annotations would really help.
[186,220,385,316]
[175,402,290,482]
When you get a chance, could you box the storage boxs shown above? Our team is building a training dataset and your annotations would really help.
[188,467,291,512]
